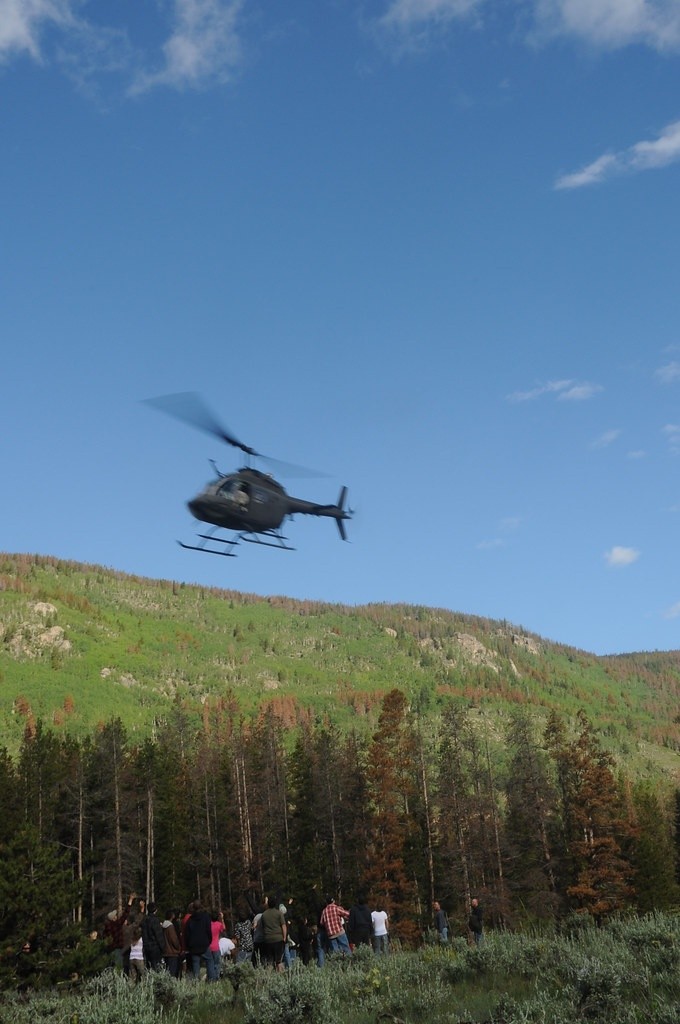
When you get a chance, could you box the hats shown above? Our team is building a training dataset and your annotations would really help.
[279,903,287,914]
[107,909,117,920]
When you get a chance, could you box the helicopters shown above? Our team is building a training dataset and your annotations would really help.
[139,387,353,557]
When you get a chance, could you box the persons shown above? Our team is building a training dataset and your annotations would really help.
[220,482,249,507]
[0,885,307,990]
[304,880,336,975]
[349,896,374,955]
[468,897,485,948]
[322,896,356,958]
[432,898,449,950]
[367,904,393,955]
